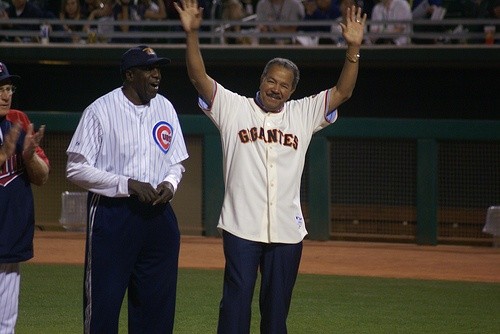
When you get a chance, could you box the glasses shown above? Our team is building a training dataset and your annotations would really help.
[0,86,16,95]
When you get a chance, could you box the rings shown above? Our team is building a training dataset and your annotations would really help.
[356,19,361,23]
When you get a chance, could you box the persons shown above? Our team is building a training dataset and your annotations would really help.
[0,0,500,45]
[65,46,190,334]
[170,0,367,334]
[0,62,52,334]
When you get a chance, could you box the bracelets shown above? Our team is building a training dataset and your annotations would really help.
[345,51,360,63]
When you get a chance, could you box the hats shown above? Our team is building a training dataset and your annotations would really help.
[0,62,21,83]
[120,45,170,70]
